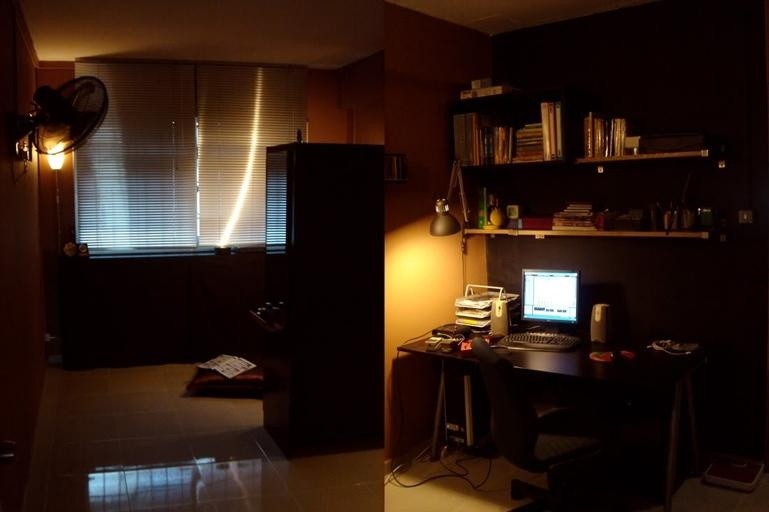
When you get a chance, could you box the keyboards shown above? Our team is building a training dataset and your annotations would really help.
[504,331,579,349]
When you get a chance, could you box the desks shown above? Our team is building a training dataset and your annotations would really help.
[395,321,710,512]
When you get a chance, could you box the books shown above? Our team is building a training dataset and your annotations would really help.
[431,323,472,339]
[199,353,258,379]
[451,84,706,232]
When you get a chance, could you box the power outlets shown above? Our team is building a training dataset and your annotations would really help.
[44,332,52,341]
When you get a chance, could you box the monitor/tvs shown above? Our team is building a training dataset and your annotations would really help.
[518,267,581,331]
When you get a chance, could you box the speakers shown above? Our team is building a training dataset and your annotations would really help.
[590,304,614,343]
[491,299,509,334]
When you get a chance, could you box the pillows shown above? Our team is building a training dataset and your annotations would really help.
[186,351,266,403]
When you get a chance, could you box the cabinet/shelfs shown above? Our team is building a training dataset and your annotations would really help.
[250,139,389,462]
[456,135,718,243]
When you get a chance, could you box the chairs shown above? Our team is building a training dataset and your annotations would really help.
[483,367,656,510]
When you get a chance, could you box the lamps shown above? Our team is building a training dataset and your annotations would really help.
[426,156,474,299]
[44,140,70,359]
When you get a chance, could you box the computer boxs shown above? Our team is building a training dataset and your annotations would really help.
[437,362,487,447]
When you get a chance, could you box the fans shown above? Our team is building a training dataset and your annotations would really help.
[1,75,109,164]
